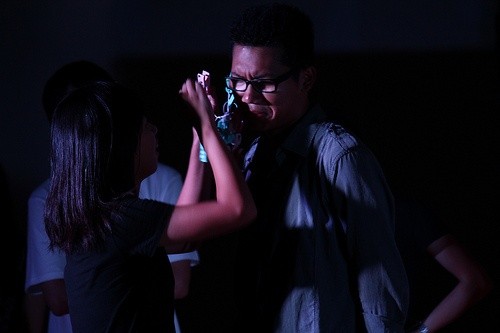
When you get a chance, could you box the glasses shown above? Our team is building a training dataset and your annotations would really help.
[225,75,293,93]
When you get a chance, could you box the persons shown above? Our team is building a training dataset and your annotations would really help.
[21,13,492,333]
[44,69,257,333]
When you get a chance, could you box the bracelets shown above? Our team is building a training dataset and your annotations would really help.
[413,322,432,333]
